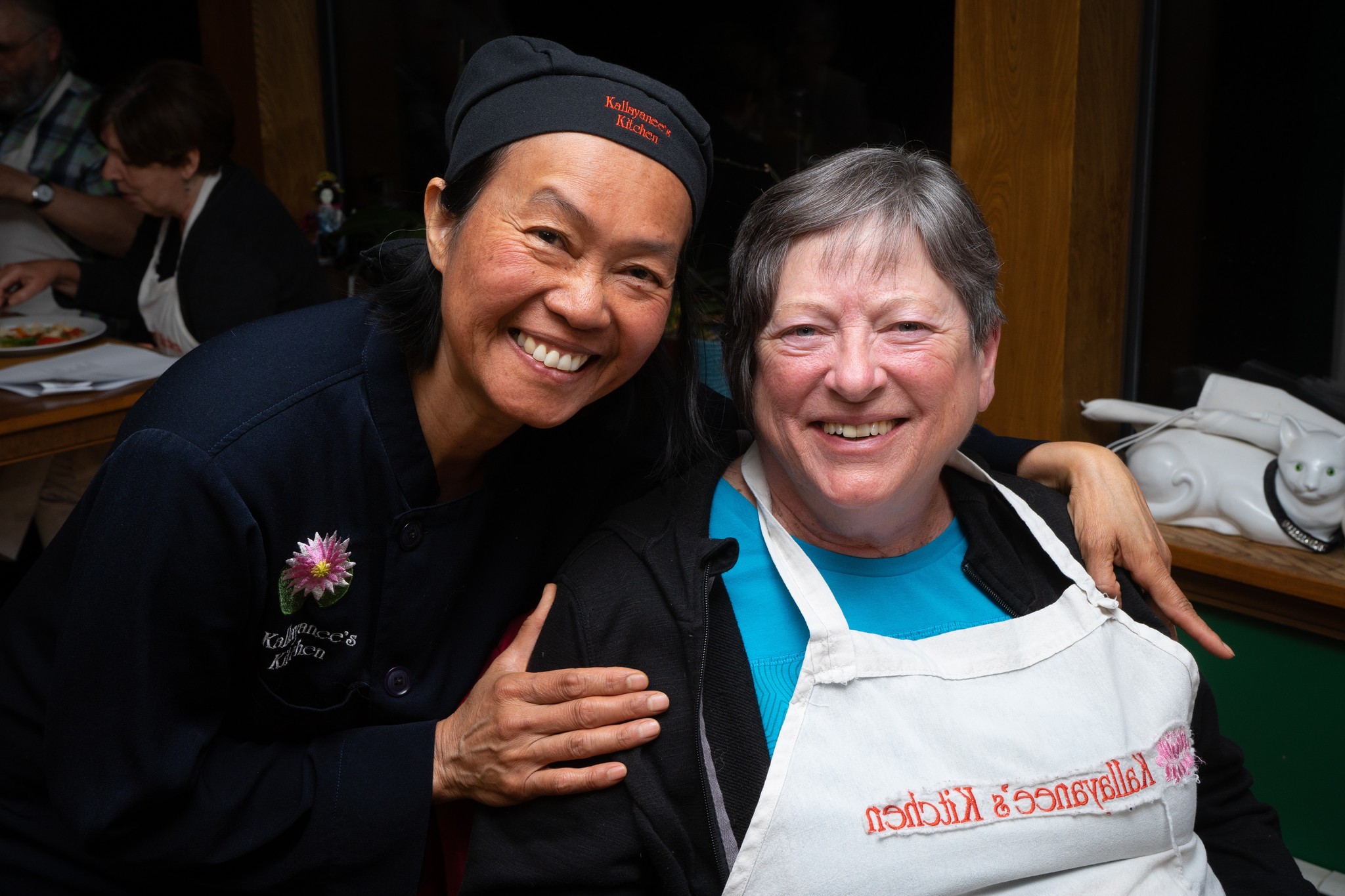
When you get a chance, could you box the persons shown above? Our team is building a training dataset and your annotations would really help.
[306,173,350,264]
[0,0,143,256]
[452,140,1323,896]
[0,36,1236,896]
[0,56,331,353]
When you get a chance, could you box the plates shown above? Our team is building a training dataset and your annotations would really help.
[0,317,106,354]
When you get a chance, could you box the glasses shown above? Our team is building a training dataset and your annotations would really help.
[0,21,51,57]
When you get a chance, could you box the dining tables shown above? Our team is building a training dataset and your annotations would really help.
[1,321,172,469]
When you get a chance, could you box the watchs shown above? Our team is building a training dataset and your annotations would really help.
[31,179,54,209]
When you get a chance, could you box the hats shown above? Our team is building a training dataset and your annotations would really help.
[443,35,714,235]
